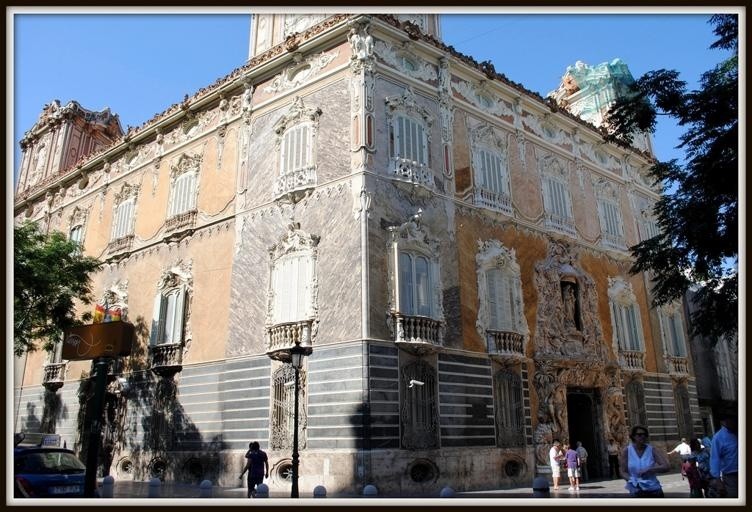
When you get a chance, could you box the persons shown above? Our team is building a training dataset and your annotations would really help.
[575,440,589,483]
[607,439,623,479]
[561,443,580,490]
[438,63,449,85]
[665,398,738,498]
[618,423,670,497]
[218,93,227,122]
[561,284,577,328]
[548,440,564,490]
[245,441,270,498]
[363,24,376,57]
[243,82,254,106]
[238,441,257,498]
[347,27,361,57]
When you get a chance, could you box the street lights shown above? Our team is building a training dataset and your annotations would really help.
[286,338,304,500]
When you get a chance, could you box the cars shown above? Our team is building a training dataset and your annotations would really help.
[13,429,87,499]
